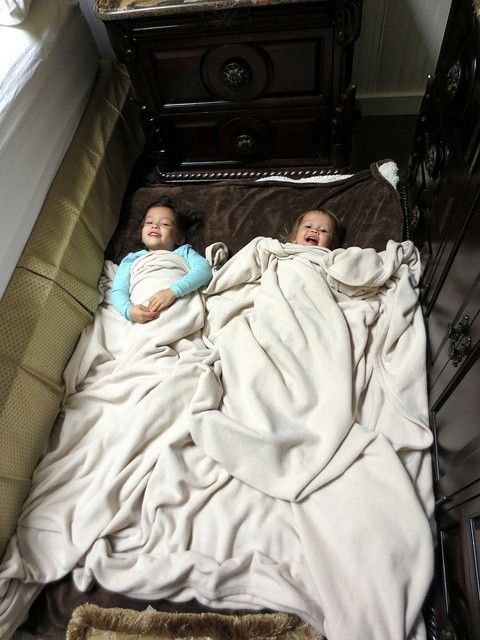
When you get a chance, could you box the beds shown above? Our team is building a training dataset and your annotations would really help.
[2,161,407,639]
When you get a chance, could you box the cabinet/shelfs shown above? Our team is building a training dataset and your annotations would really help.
[94,0,356,170]
[409,1,480,317]
[433,148,479,507]
[430,477,479,640]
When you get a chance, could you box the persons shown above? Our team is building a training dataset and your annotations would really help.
[278,207,343,251]
[110,195,213,322]
